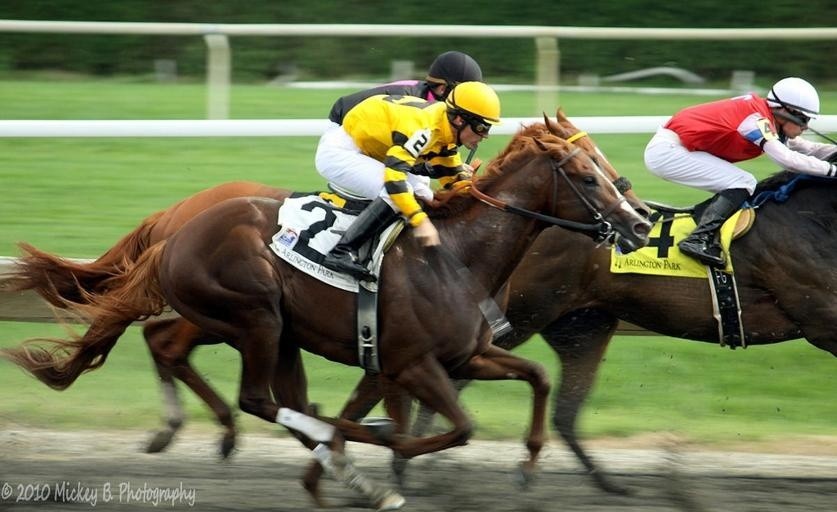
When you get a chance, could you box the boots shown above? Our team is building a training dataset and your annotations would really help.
[678,195,739,270]
[323,196,397,282]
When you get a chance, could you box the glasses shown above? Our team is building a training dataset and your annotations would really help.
[466,118,492,138]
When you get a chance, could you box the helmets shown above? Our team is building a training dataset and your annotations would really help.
[425,51,483,86]
[445,81,503,126]
[766,77,820,119]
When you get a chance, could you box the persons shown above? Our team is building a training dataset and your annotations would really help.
[314,80,500,283]
[642,73,837,264]
[323,50,481,136]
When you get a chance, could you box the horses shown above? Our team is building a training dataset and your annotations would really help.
[408,150,837,498]
[0,105,655,512]
